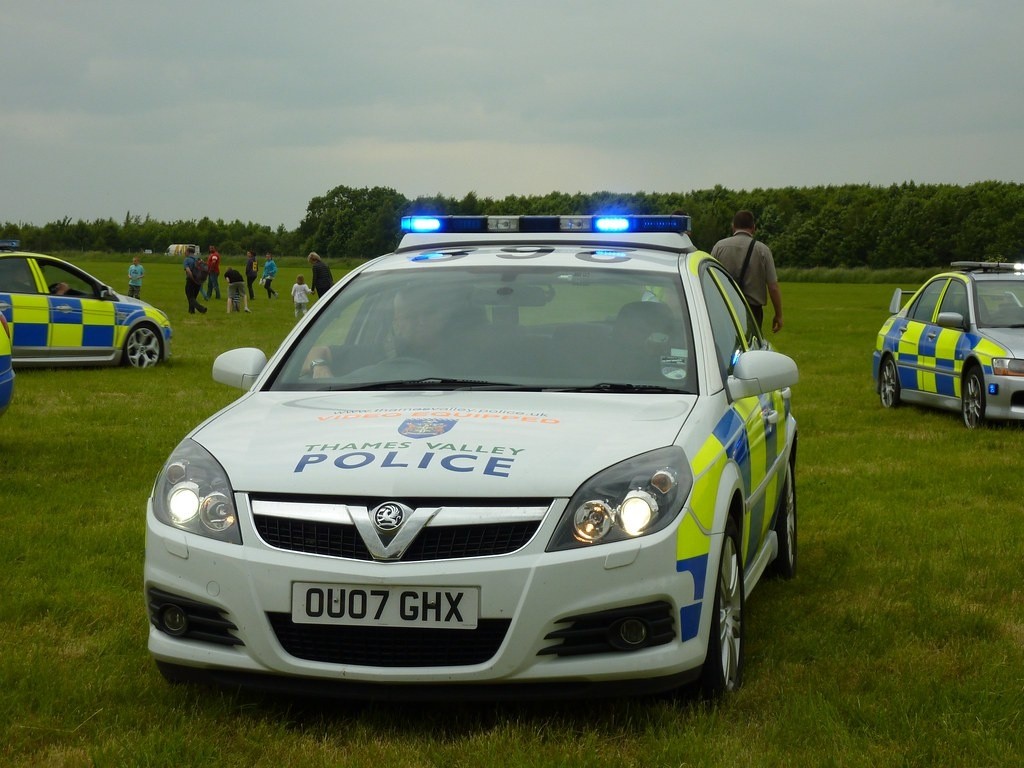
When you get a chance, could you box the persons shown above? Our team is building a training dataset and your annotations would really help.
[184,243,220,313]
[128,256,145,299]
[246,249,258,300]
[711,211,783,333]
[262,253,278,299]
[291,251,334,317]
[56,282,68,295]
[224,266,252,313]
[300,284,442,379]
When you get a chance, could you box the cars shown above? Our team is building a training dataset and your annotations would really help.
[872,260,1024,431]
[140,211,801,697]
[0,240,173,369]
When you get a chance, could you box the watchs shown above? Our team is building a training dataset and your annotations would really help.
[310,358,326,370]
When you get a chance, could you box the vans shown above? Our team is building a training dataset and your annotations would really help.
[165,244,201,256]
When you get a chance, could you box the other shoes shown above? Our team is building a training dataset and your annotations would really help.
[295,312,298,317]
[226,311,232,313]
[245,307,251,313]
[187,312,195,316]
[215,298,220,300]
[250,297,256,301]
[274,293,279,298]
[204,298,209,302]
[201,308,207,314]
[268,298,271,300]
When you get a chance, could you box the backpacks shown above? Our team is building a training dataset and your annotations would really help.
[190,256,208,282]
